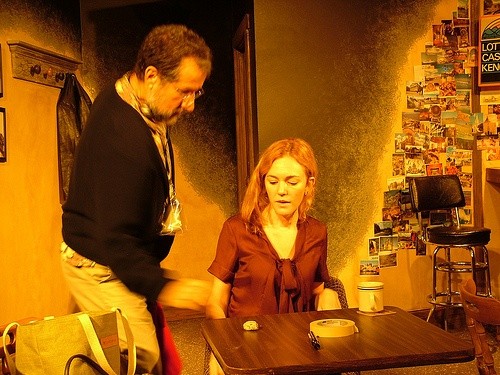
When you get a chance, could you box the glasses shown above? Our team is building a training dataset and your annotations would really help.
[175,88,206,108]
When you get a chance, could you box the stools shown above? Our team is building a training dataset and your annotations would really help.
[409,174,492,332]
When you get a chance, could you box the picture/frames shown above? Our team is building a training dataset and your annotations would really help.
[0,107,7,162]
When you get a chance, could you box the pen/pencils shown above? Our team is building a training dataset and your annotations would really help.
[306,331,320,349]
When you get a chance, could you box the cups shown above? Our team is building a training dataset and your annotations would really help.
[356,282,386,313]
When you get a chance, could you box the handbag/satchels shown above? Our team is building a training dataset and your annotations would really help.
[2,306,137,375]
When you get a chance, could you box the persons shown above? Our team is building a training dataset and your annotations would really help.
[208,138,330,375]
[59,25,217,375]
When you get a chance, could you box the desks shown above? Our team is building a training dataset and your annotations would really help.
[201,306,476,375]
